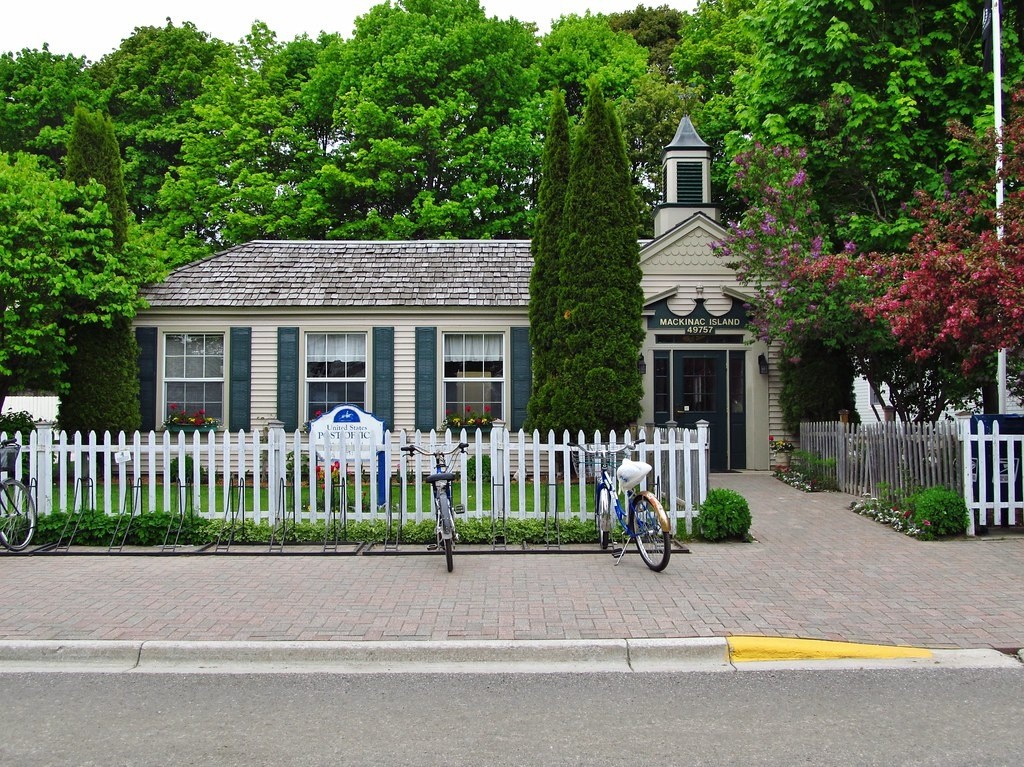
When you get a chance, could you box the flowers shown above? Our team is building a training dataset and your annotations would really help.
[160,404,221,434]
[769,436,795,451]
[303,411,322,434]
[438,406,497,436]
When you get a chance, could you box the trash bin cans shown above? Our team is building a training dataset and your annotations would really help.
[968,412,1024,502]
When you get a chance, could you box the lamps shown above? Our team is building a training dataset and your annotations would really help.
[637,352,646,375]
[758,353,769,374]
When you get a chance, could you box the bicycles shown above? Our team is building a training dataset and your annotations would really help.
[399,442,471,572]
[0,438,37,551]
[564,439,673,573]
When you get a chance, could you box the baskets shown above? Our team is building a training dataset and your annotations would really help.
[571,442,632,477]
[0,443,21,471]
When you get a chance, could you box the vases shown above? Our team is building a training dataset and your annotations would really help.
[773,449,792,467]
[446,424,492,433]
[162,423,218,432]
